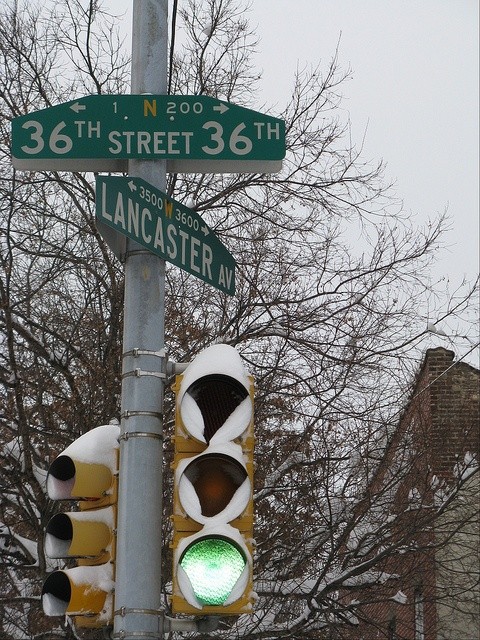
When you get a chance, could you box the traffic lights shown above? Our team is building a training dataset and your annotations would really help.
[174,362,254,615]
[40,442,117,627]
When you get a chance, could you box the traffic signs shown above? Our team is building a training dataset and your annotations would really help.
[96,175,236,295]
[12,94,286,172]
